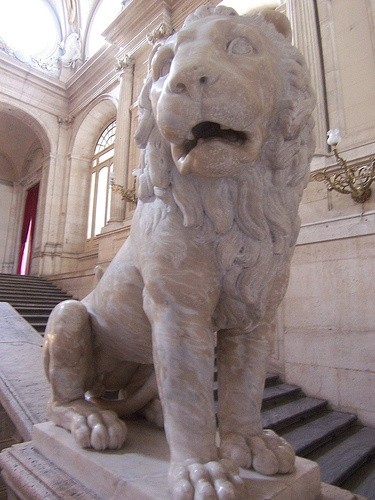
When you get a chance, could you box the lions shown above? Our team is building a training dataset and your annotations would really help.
[41,4,317,500]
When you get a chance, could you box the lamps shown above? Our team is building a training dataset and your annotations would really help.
[310,129,375,203]
[109,168,140,205]
